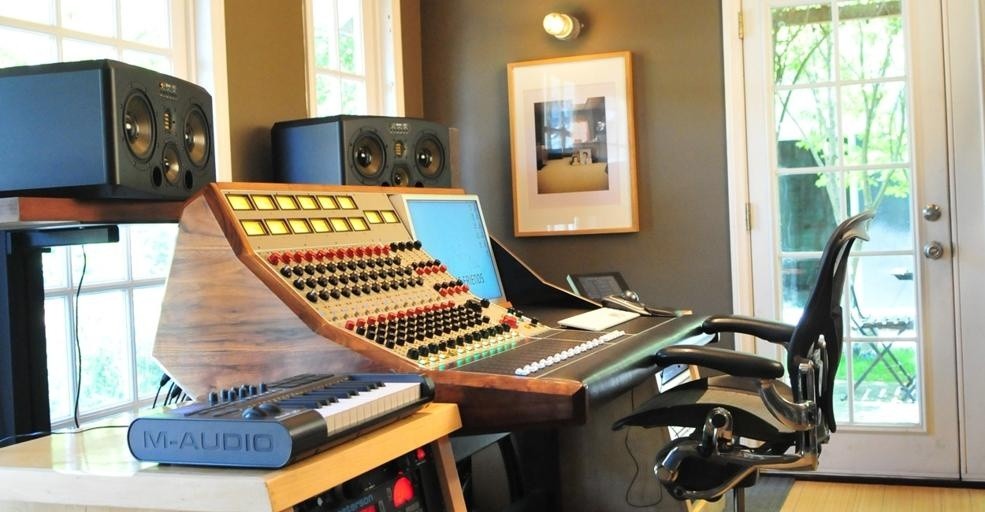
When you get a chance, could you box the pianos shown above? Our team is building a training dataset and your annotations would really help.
[127,372,435,469]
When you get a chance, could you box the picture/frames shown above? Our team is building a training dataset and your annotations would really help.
[506,49,640,239]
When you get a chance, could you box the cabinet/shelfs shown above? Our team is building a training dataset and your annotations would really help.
[0,401,471,512]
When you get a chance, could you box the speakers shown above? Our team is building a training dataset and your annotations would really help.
[0,59,216,202]
[271,115,452,188]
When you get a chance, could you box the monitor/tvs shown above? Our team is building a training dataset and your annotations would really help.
[388,194,507,305]
[565,269,632,304]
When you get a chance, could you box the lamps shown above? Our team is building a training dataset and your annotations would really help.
[542,12,581,41]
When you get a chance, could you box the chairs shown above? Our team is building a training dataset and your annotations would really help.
[840,285,919,404]
[613,210,875,512]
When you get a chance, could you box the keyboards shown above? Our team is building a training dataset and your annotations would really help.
[557,307,640,333]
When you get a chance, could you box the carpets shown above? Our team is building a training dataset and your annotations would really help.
[724,474,796,512]
